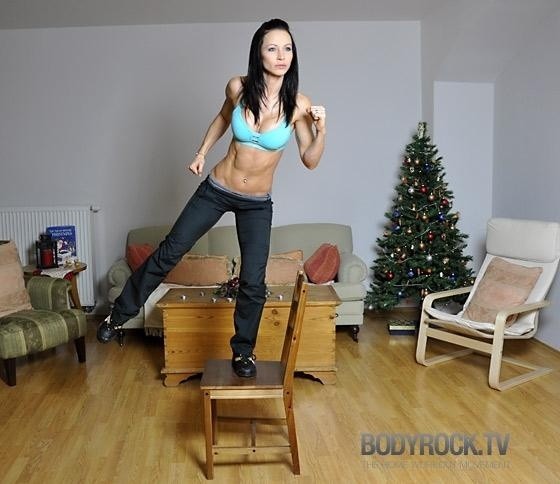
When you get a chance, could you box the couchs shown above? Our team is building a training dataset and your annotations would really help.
[106,221,368,347]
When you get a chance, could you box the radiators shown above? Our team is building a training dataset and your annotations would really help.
[0,204,104,308]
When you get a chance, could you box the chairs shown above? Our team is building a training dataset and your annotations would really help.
[413,213,559,393]
[0,238,89,387]
[198,267,309,481]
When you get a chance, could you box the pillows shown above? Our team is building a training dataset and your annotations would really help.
[279,250,304,261]
[231,255,306,284]
[126,243,155,273]
[1,241,34,321]
[161,253,231,286]
[303,243,340,285]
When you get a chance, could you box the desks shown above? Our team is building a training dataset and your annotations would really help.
[25,261,89,313]
[154,283,343,388]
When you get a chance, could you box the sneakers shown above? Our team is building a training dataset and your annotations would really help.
[230,352,259,378]
[97,312,123,344]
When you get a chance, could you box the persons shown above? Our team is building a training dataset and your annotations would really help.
[96,19,325,378]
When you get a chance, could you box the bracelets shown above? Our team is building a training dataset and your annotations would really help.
[195,153,205,156]
